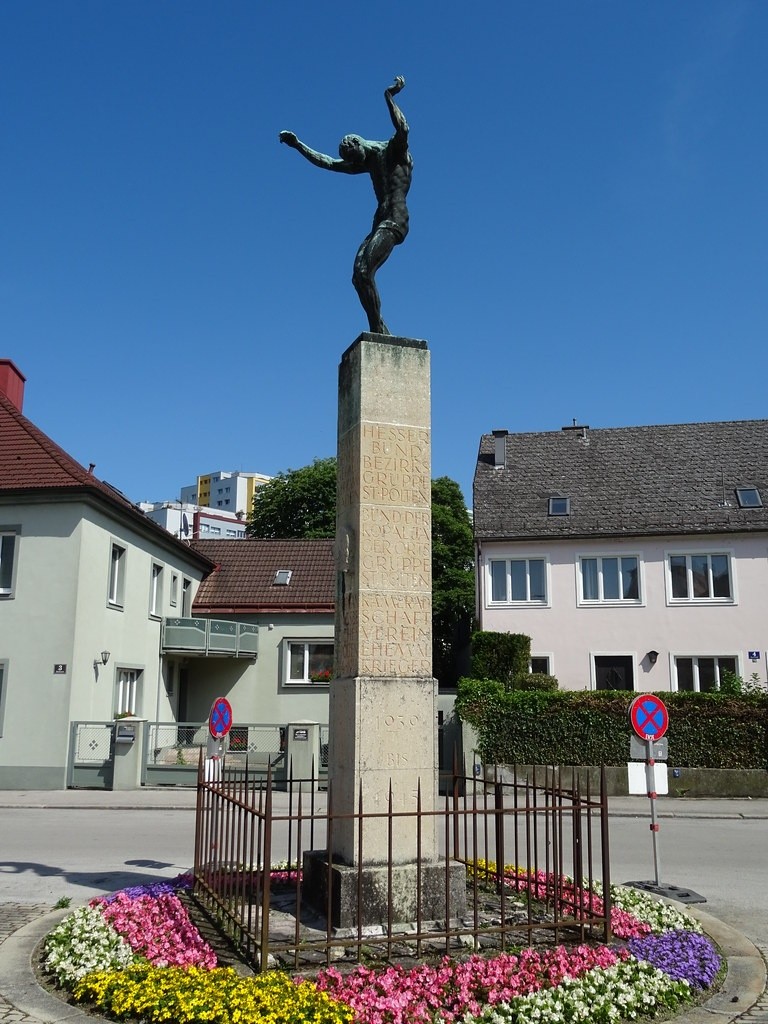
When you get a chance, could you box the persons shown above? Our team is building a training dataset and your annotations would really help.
[278,75,415,336]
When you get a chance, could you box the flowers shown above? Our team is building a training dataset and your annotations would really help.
[114,711,136,719]
[309,669,333,682]
[229,737,247,751]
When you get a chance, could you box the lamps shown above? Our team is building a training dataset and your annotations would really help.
[94,650,110,669]
[647,650,659,663]
